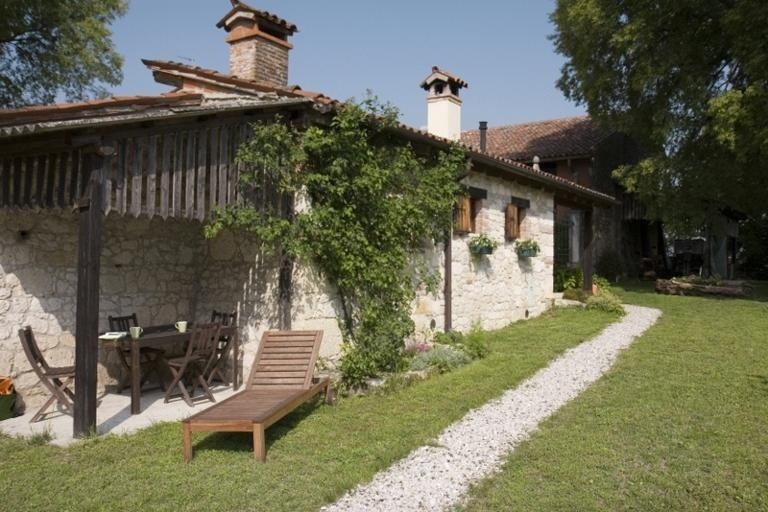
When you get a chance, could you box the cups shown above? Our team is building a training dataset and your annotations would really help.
[130,327,143,339]
[175,321,187,333]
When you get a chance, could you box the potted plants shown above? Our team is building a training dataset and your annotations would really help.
[514,238,541,257]
[466,232,497,255]
